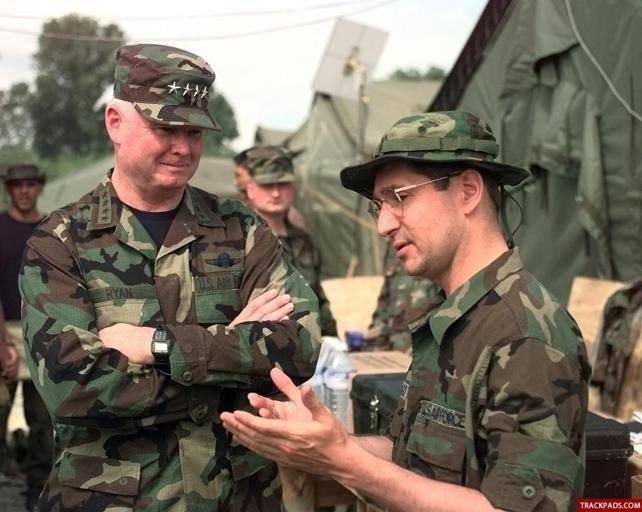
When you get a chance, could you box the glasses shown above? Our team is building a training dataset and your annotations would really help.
[367,171,462,222]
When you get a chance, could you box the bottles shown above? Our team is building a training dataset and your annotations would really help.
[323,345,357,423]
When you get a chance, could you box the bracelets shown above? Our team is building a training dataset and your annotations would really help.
[6,340,14,347]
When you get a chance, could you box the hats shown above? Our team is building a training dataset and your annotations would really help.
[340,111,529,200]
[113,44,223,131]
[246,147,295,185]
[0,165,45,184]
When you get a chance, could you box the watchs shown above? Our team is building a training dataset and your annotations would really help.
[151,328,172,372]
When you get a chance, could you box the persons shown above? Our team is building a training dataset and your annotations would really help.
[0,305,22,393]
[221,110,591,511]
[243,147,339,512]
[233,147,307,232]
[18,43,321,511]
[1,163,49,511]
[361,255,446,358]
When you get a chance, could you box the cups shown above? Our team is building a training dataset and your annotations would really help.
[345,330,364,352]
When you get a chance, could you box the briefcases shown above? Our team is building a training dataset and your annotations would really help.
[349,372,634,499]
